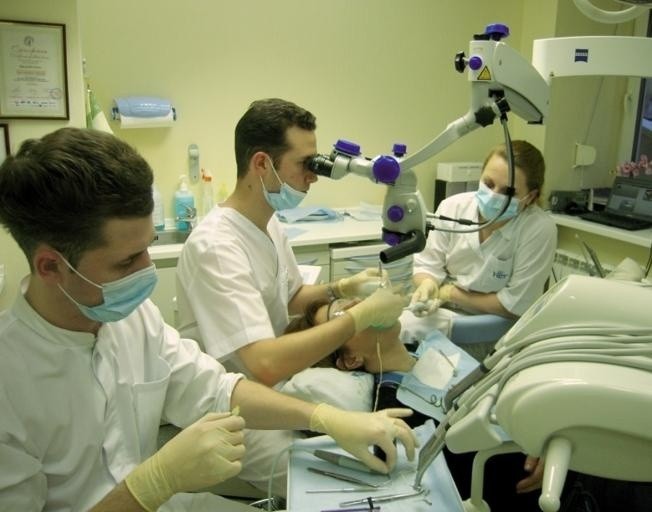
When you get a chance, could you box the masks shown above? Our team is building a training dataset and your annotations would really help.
[257,155,310,213]
[474,179,532,223]
[45,247,161,323]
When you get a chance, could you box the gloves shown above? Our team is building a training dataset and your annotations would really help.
[337,266,394,301]
[121,410,248,512]
[344,287,406,347]
[307,402,422,476]
[408,276,457,320]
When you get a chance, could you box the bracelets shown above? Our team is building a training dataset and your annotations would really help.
[325,284,336,303]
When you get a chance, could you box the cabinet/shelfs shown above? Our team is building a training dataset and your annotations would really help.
[291,242,393,289]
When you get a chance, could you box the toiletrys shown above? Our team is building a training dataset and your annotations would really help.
[174,175,193,231]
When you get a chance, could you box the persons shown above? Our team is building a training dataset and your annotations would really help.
[281,294,652,511]
[175,96,409,502]
[405,139,560,325]
[0,124,422,511]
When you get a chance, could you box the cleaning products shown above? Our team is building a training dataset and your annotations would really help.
[196,169,214,225]
[152,185,164,230]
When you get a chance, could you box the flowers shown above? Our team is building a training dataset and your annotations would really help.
[609,153,651,178]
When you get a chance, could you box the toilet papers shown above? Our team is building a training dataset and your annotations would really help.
[116,109,173,130]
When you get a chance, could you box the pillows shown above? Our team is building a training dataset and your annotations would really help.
[277,304,475,434]
